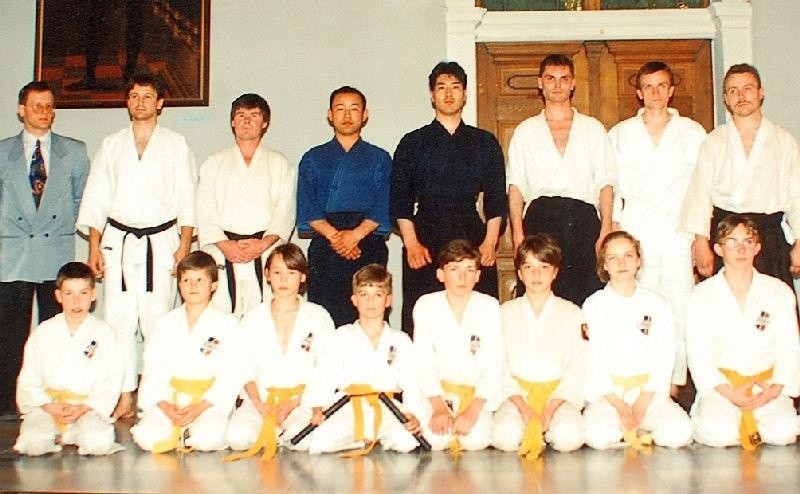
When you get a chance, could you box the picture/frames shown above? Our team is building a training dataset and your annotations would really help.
[33,0,211,109]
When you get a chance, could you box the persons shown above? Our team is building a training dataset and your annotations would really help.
[505,55,614,309]
[676,63,798,292]
[490,233,589,452]
[389,62,509,344]
[74,71,197,421]
[0,82,90,422]
[686,213,800,447]
[302,263,427,454]
[409,238,506,451]
[196,94,298,323]
[128,251,243,453]
[609,62,708,415]
[79,2,144,86]
[297,85,394,330]
[582,231,693,450]
[13,262,126,457]
[226,243,336,451]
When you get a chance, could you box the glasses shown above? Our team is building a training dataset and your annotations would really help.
[32,105,57,114]
[719,239,756,252]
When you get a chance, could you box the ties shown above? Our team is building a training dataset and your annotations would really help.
[29,139,48,208]
